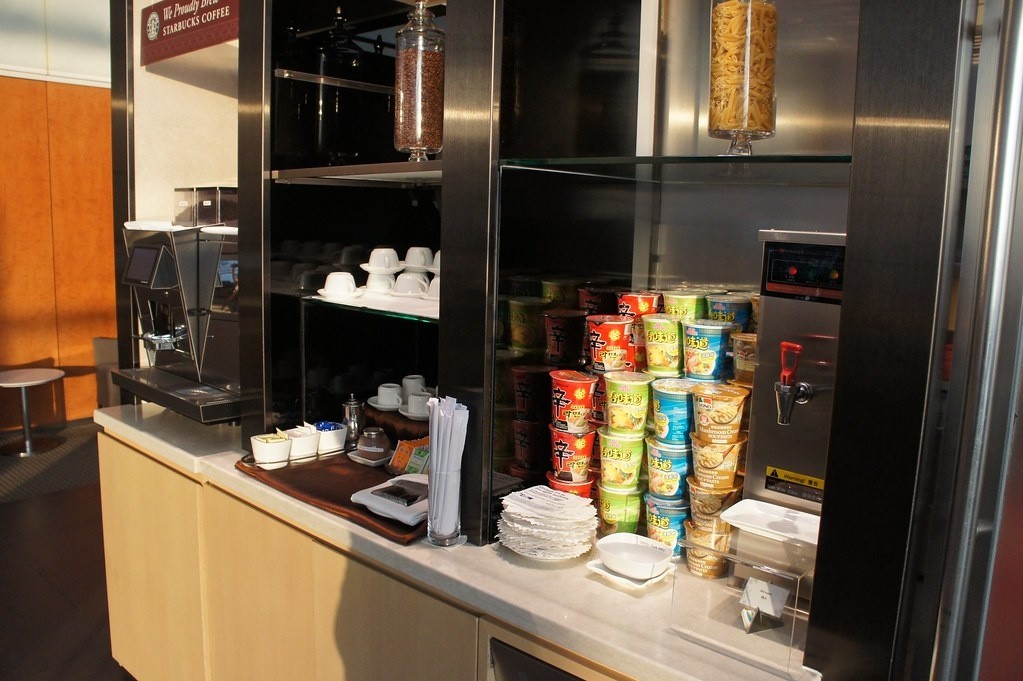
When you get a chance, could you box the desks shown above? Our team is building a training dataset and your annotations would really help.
[0,368,65,457]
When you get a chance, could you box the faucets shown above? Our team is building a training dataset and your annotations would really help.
[773,339,801,425]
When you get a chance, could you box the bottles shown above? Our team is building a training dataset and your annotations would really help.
[356,427,390,461]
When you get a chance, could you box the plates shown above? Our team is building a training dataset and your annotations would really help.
[367,396,407,411]
[347,449,394,467]
[425,267,440,276]
[425,387,436,395]
[719,499,821,546]
[360,263,405,274]
[585,557,679,589]
[399,407,429,420]
[317,286,439,301]
[400,260,429,273]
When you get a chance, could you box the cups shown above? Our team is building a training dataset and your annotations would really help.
[408,392,432,416]
[433,250,440,267]
[367,248,401,269]
[426,465,462,547]
[324,272,357,295]
[402,375,427,404]
[404,247,434,267]
[366,274,395,291]
[392,273,440,298]
[377,384,403,406]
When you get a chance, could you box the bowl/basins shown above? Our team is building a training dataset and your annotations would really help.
[596,533,673,580]
[250,421,348,469]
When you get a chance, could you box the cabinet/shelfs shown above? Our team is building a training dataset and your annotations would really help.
[94,0,1023,681]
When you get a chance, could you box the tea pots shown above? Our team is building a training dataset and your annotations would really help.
[341,393,366,451]
